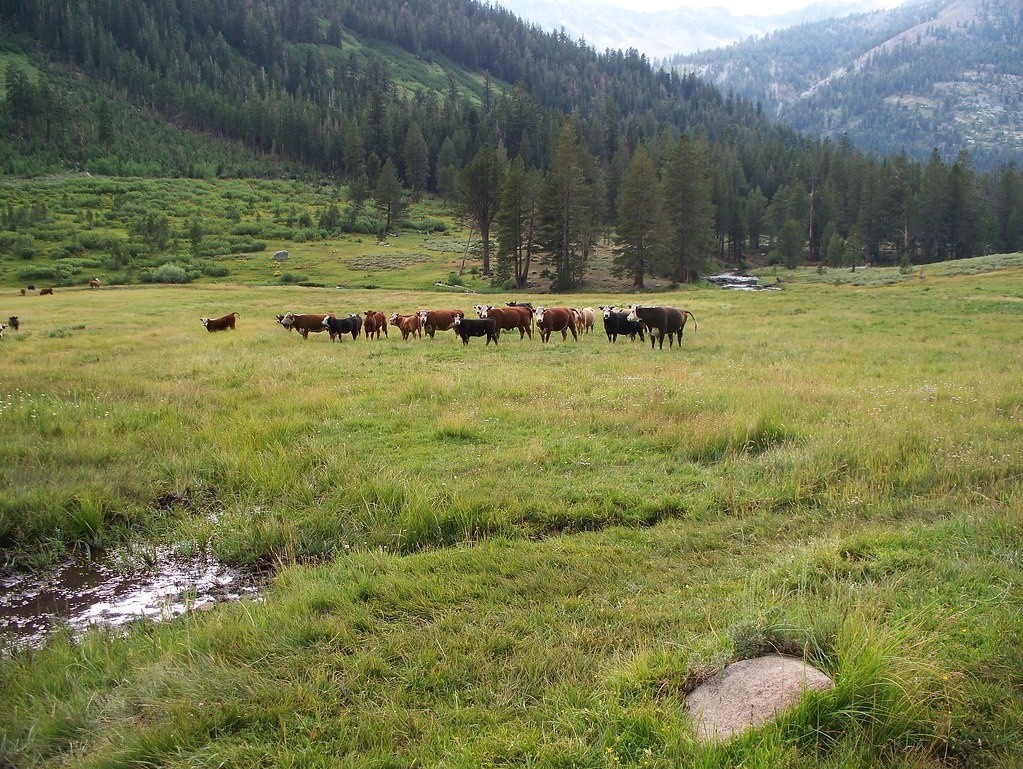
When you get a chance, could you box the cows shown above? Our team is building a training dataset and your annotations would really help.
[90,277,101,289]
[27,285,36,290]
[389,309,464,341]
[321,315,358,342]
[599,304,698,350]
[363,310,388,340]
[40,289,53,296]
[451,313,498,346]
[0,325,8,337]
[280,312,337,340]
[8,316,19,330]
[473,300,535,340]
[348,313,363,337]
[200,312,241,334]
[275,314,294,331]
[21,289,26,295]
[532,306,596,344]
